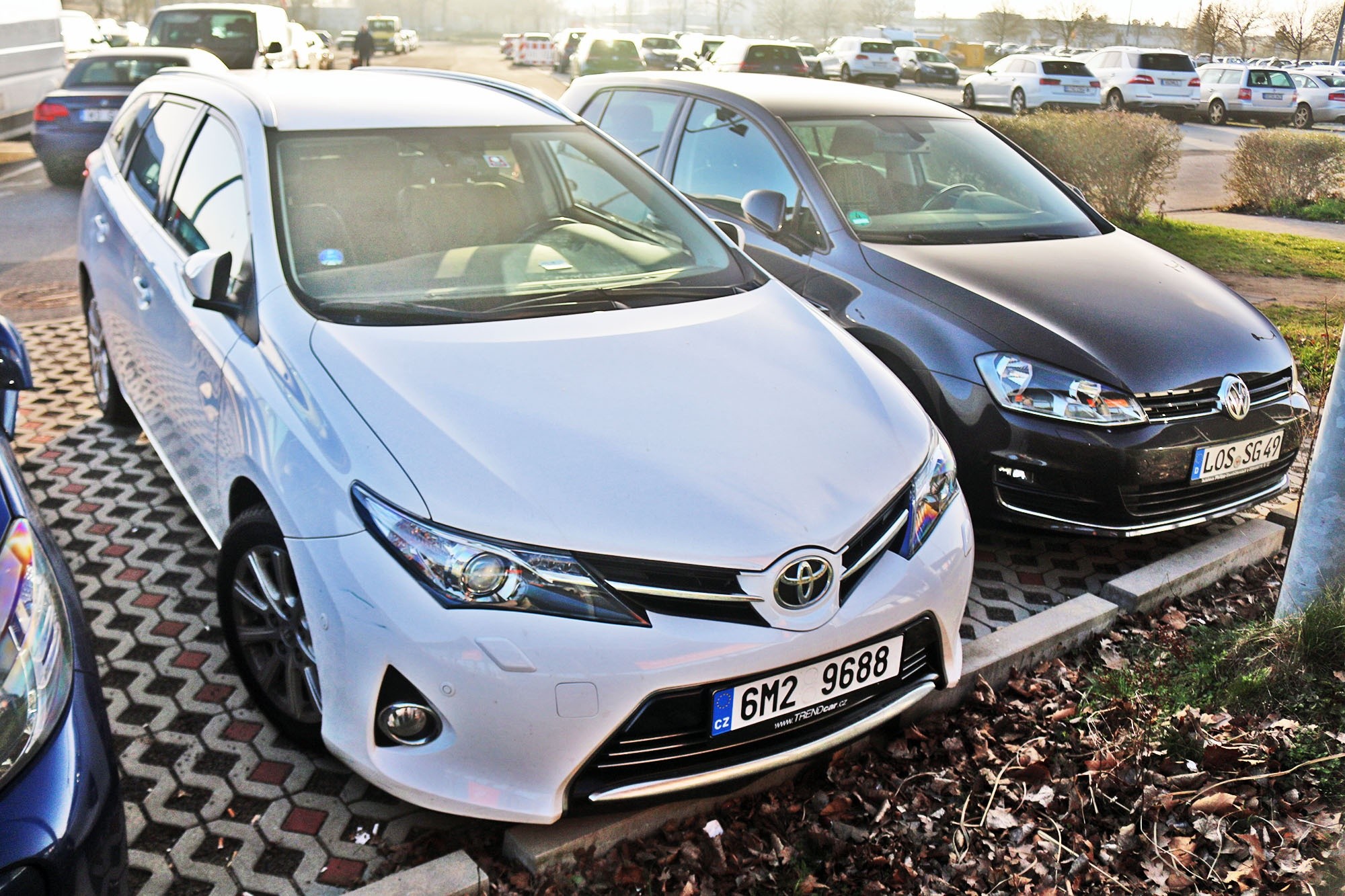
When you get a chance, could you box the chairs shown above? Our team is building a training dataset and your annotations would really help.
[286,202,357,275]
[816,126,895,217]
[393,184,526,256]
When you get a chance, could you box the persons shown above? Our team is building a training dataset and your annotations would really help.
[355,25,376,66]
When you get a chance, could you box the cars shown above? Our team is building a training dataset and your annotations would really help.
[62,10,149,67]
[1194,63,1298,127]
[962,53,1103,114]
[668,33,822,78]
[290,24,338,69]
[550,29,645,80]
[984,42,1084,56]
[1190,54,1345,69]
[894,47,961,85]
[624,35,683,70]
[0,315,129,896]
[30,46,230,185]
[1266,70,1345,129]
[75,65,974,826]
[543,73,1309,539]
[335,32,359,50]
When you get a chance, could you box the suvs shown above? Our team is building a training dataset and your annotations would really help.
[817,37,901,88]
[1085,44,1202,124]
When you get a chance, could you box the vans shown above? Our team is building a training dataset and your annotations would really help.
[136,2,298,80]
[366,16,400,54]
